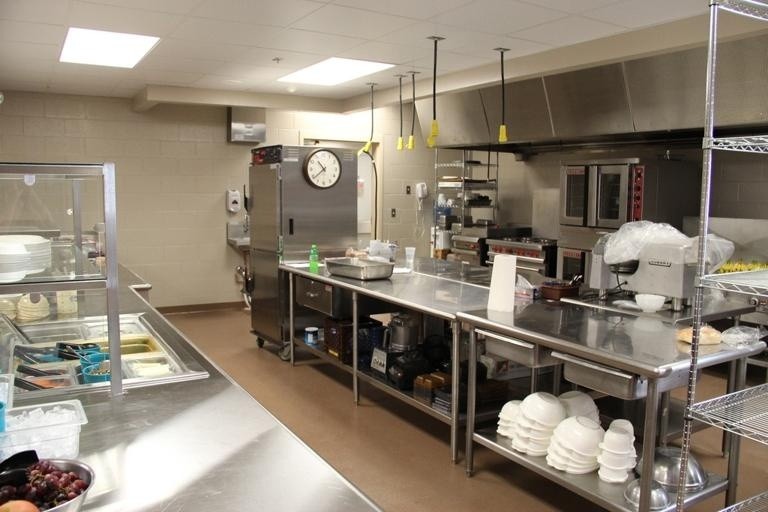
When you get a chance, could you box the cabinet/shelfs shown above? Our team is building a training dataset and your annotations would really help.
[433,148,498,248]
[677,0,768,512]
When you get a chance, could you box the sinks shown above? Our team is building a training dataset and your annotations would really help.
[227,237,251,251]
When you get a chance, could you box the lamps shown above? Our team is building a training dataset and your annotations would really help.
[361,35,510,151]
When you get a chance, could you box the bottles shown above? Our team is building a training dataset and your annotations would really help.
[307,241,319,276]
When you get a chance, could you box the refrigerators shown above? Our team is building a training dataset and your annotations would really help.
[250,144,360,358]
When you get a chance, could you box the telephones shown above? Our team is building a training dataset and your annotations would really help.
[417,183,428,198]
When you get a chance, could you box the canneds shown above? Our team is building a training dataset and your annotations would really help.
[305,326,319,346]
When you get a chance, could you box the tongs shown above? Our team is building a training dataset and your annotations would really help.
[12,345,57,364]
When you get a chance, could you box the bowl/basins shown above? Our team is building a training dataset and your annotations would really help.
[37,459,94,512]
[496,390,637,483]
[623,447,706,510]
[633,294,666,313]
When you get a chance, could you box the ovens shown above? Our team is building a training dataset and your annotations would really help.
[557,155,684,284]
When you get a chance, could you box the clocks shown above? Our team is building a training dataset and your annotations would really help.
[303,148,342,189]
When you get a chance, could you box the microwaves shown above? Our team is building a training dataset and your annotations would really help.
[429,227,454,259]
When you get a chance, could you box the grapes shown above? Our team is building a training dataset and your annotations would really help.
[0,460,88,512]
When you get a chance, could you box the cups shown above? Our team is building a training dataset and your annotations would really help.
[405,247,416,263]
[304,327,318,345]
